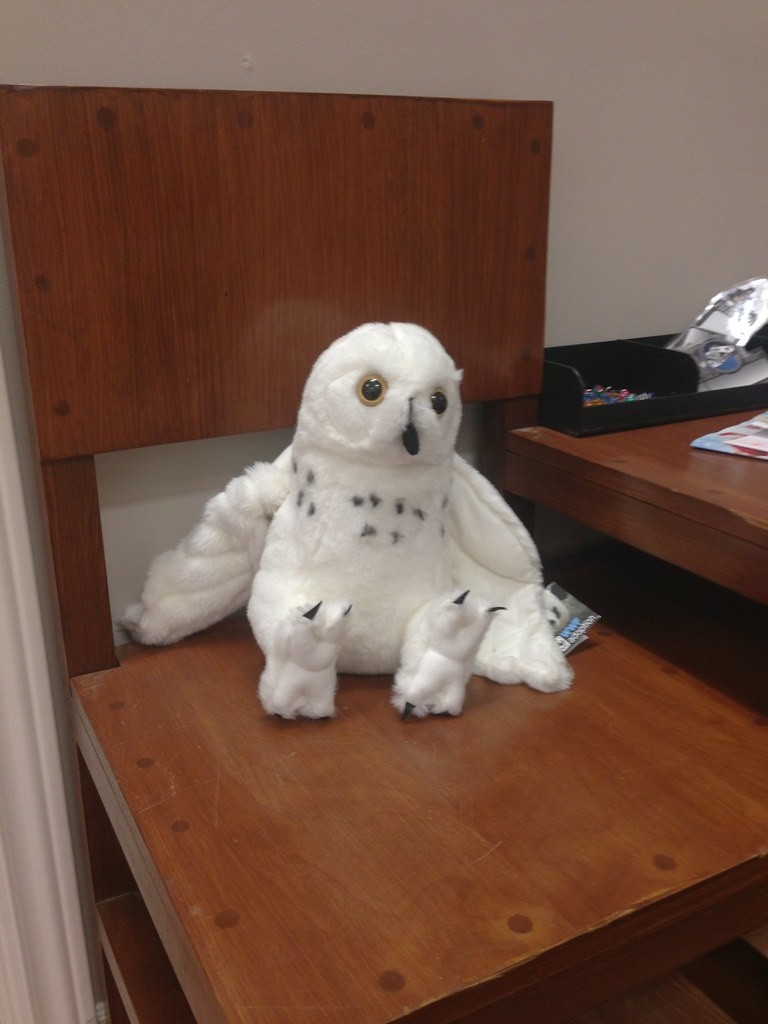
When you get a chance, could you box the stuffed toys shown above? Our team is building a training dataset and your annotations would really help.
[119,321,574,720]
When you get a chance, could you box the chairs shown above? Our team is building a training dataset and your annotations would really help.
[0,83,768,1024]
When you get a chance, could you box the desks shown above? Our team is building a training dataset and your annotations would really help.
[497,407,768,725]
